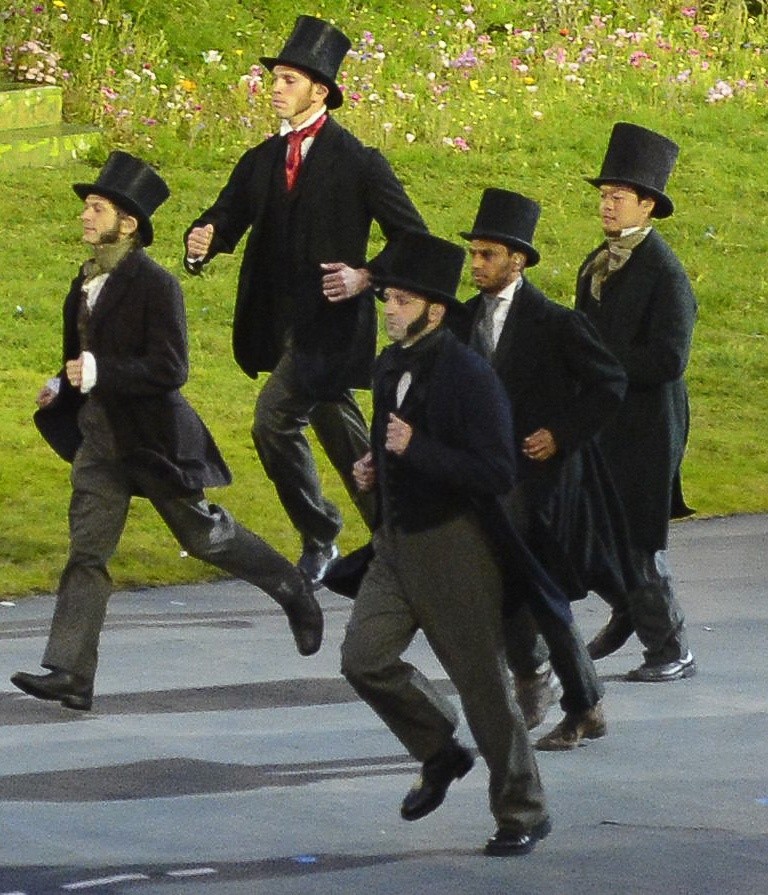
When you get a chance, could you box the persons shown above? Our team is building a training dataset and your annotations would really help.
[586,122,699,683]
[444,186,629,754]
[337,226,554,856]
[184,14,431,587]
[8,149,325,712]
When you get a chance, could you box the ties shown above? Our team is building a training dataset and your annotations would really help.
[479,280,523,362]
[581,227,650,301]
[76,261,112,353]
[285,112,328,191]
[382,350,411,415]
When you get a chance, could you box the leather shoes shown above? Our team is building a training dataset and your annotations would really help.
[10,670,93,712]
[585,613,635,662]
[294,543,342,591]
[397,741,476,822]
[625,647,698,682]
[484,817,552,856]
[284,564,325,657]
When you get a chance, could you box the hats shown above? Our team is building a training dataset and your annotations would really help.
[582,121,681,219]
[259,15,352,111]
[72,149,170,247]
[458,186,543,269]
[366,225,467,317]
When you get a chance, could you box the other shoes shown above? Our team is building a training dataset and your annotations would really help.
[534,705,609,752]
[514,669,564,731]
